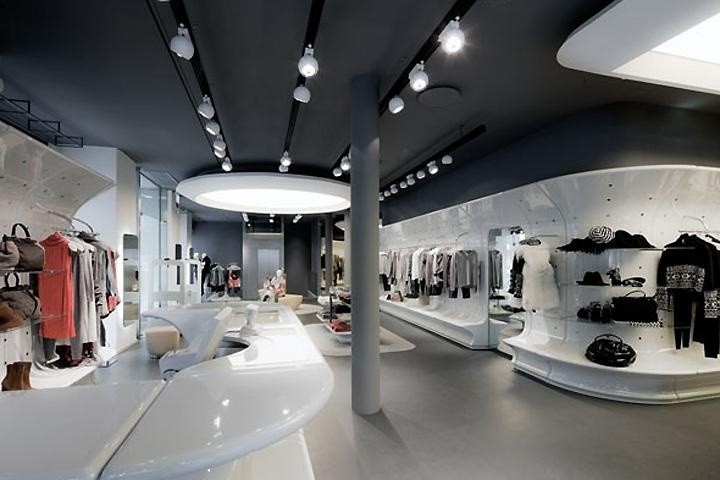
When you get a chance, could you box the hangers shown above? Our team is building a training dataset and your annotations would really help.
[388,241,467,250]
[678,230,720,236]
[49,226,101,238]
[519,234,559,246]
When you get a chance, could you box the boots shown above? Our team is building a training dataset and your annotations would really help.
[14,362,33,389]
[56,345,78,366]
[69,345,83,362]
[3,364,25,390]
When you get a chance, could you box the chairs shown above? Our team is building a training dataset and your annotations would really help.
[278,294,303,310]
[159,306,234,380]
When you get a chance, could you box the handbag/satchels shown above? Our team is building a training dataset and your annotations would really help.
[587,334,636,367]
[0,223,46,271]
[1,272,41,319]
[613,290,658,322]
[576,302,612,322]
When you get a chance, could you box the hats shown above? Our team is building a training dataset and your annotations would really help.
[577,271,610,286]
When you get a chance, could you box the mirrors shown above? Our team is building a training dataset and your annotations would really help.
[123,234,139,328]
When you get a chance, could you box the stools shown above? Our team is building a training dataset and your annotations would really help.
[146,326,181,359]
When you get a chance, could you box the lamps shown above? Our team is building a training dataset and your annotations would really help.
[214,149,227,158]
[389,98,405,113]
[340,161,351,171]
[407,178,416,185]
[198,103,215,119]
[391,188,398,193]
[280,156,291,166]
[222,162,232,171]
[400,183,408,188]
[417,170,425,179]
[213,140,226,151]
[297,56,319,77]
[429,165,439,174]
[441,154,453,165]
[335,169,342,176]
[206,122,220,135]
[384,190,391,197]
[441,30,465,54]
[294,87,311,103]
[410,70,429,91]
[278,165,288,172]
[379,196,384,201]
[170,36,195,60]
